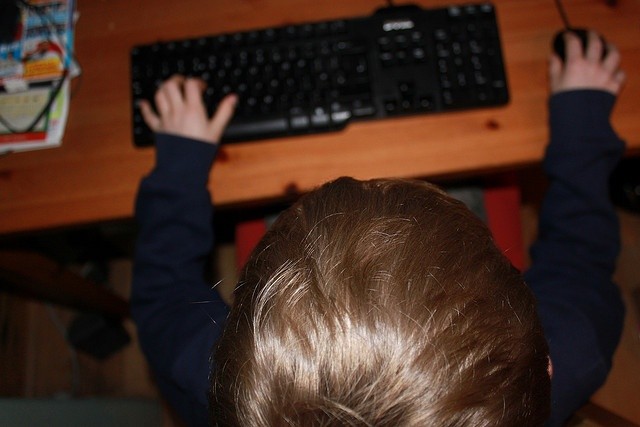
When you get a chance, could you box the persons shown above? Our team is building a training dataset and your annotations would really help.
[127,29,626,425]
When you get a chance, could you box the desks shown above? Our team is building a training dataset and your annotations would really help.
[1,1,640,319]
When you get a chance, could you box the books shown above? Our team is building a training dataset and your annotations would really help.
[0,0,81,154]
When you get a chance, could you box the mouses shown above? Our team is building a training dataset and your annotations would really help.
[553,26,607,62]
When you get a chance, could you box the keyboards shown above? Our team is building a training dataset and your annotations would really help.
[129,3,508,147]
